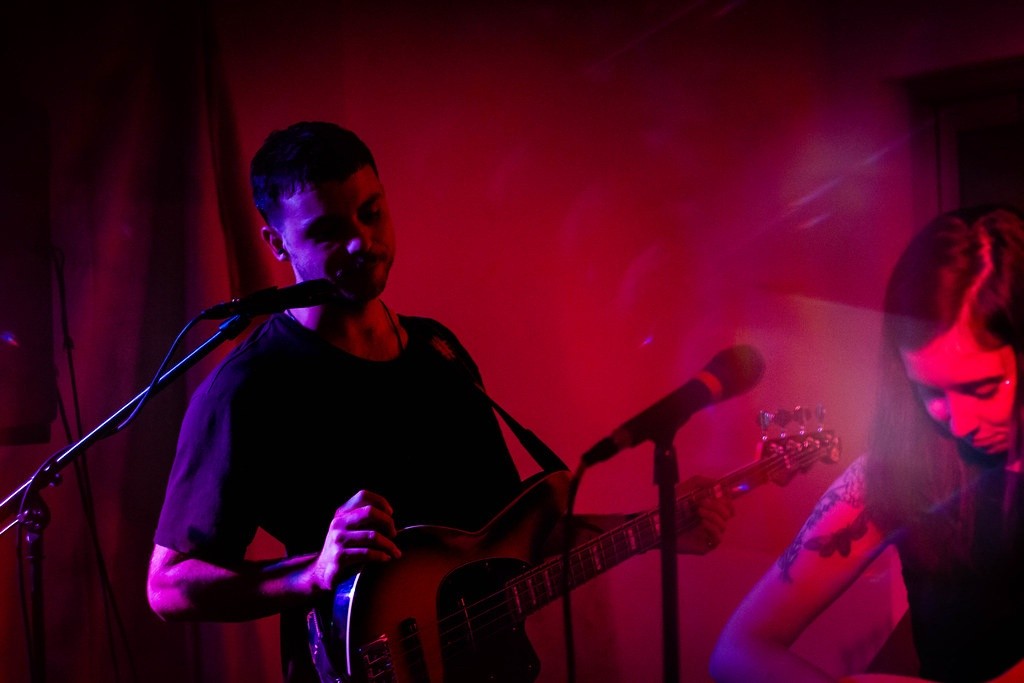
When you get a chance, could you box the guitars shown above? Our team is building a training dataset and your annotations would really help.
[300,403,843,683]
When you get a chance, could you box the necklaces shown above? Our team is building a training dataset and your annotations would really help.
[287,296,404,357]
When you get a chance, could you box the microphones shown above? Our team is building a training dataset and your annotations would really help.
[199,280,335,321]
[581,346,766,469]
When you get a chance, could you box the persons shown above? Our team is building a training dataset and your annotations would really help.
[708,205,1024,683]
[144,119,738,683]
